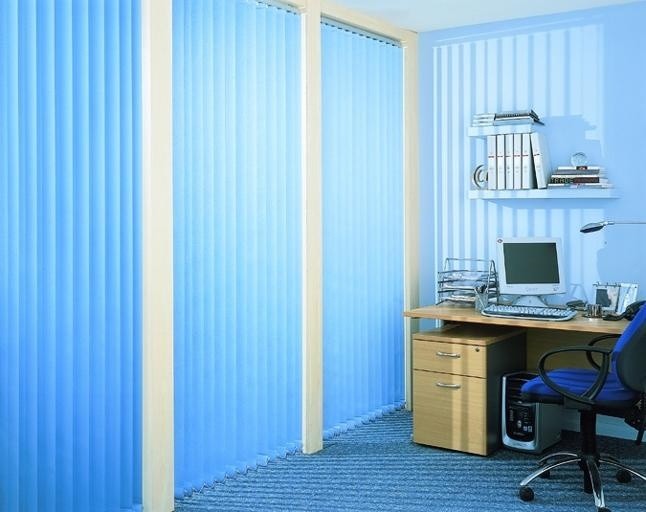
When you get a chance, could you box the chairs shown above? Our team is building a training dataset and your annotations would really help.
[518,304,646,511]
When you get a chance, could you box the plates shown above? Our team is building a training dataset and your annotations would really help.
[583,313,605,319]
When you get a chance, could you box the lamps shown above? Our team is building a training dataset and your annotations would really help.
[580,218,646,234]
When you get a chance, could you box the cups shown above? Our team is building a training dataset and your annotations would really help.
[587,304,604,316]
[475,292,489,312]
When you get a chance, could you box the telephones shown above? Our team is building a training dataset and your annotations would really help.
[620,300,646,321]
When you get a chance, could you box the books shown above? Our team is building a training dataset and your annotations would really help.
[469,108,548,189]
[548,163,613,188]
[442,274,485,303]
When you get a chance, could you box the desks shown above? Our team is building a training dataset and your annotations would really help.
[403,303,646,446]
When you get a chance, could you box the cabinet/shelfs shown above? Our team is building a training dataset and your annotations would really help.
[411,332,527,456]
[465,123,620,199]
[435,257,502,311]
